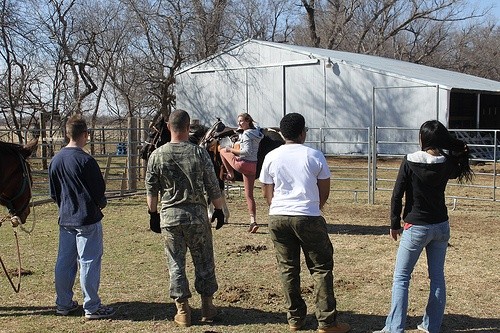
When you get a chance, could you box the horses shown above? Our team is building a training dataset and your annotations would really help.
[0,137,39,225]
[140,117,286,223]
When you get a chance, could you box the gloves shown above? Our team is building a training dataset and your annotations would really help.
[211,208,225,230]
[148,210,161,234]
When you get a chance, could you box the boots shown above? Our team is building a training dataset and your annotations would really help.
[201,294,217,320]
[174,299,191,327]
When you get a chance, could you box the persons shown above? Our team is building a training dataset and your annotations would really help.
[144,110,225,327]
[260,113,351,333]
[49,115,117,319]
[373,120,473,333]
[222,114,264,233]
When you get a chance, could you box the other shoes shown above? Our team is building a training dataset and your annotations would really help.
[290,323,302,329]
[417,320,429,333]
[319,321,352,333]
[247,222,259,233]
[224,173,236,181]
[85,304,114,319]
[55,300,78,316]
[373,328,385,333]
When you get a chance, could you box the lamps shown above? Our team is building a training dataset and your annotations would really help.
[326,58,334,69]
